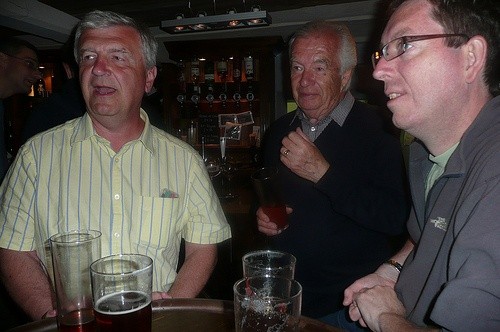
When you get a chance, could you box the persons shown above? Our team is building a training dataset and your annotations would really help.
[342,0,499,331]
[1,11,232,321]
[254,18,412,332]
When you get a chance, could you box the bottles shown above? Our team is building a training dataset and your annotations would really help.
[34,72,46,98]
[176,56,256,108]
[5,120,16,165]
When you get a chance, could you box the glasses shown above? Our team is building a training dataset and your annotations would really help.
[371,33,470,69]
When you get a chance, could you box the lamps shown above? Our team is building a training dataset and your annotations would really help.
[160,0,272,35]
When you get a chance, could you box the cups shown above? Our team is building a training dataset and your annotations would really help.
[251,165,290,231]
[49,231,101,332]
[233,275,303,332]
[242,249,296,277]
[90,254,153,332]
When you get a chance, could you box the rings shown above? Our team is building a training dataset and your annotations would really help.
[282,149,290,156]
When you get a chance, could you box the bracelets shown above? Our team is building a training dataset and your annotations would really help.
[384,257,403,271]
